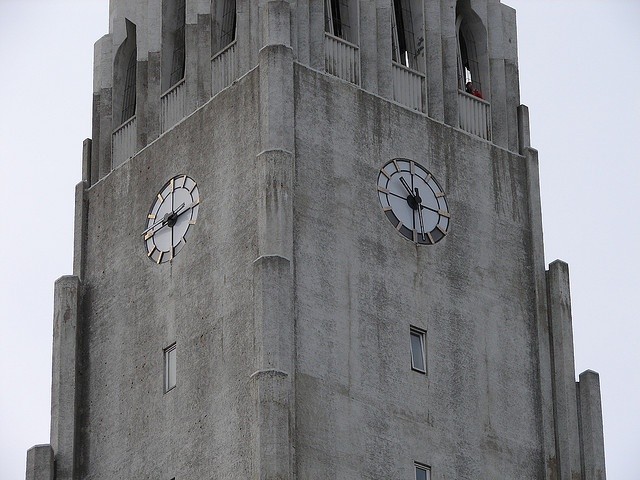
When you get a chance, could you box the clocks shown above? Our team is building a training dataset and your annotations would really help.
[376,159,451,245]
[139,174,200,265]
